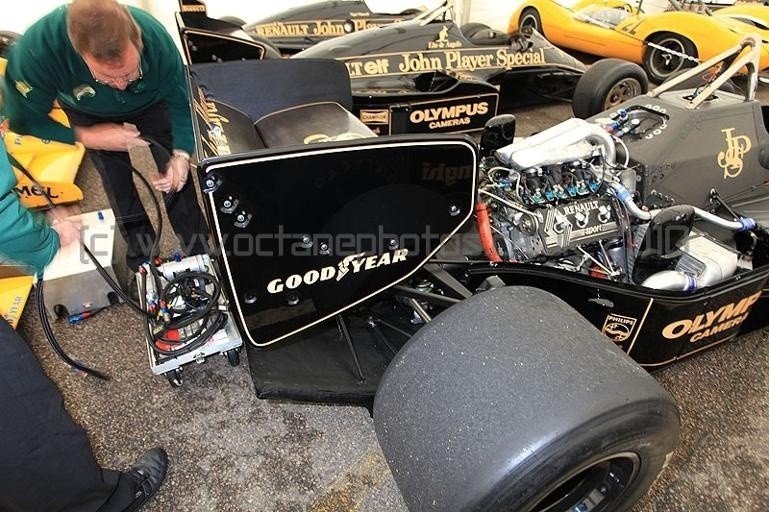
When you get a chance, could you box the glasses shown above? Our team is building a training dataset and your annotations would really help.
[92,69,145,86]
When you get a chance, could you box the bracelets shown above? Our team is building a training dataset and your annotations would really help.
[172,148,190,160]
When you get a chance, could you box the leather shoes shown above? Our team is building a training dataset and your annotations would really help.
[124,447,168,512]
[131,277,139,300]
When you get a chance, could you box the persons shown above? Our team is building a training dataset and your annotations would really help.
[0,137,168,512]
[1,1,213,301]
